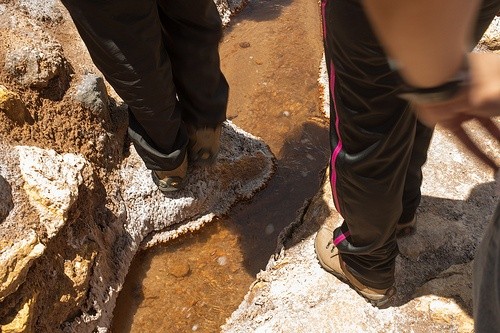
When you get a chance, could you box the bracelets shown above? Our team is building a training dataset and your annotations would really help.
[395,54,473,105]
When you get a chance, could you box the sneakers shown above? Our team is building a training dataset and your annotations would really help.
[189,128,219,165]
[314,227,397,307]
[397,213,417,237]
[152,150,189,191]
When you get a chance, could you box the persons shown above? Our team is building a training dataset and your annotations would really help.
[438,52,500,333]
[313,1,500,305]
[60,0,231,192]
